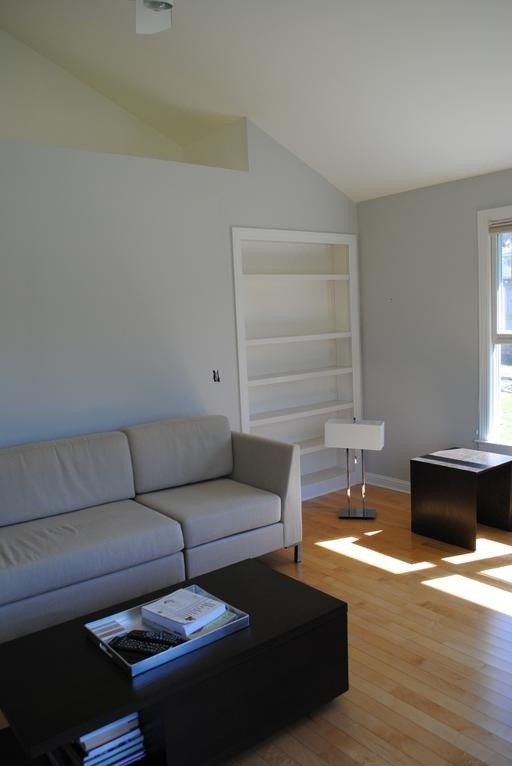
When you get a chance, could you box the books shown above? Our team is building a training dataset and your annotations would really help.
[59,709,148,766]
[140,610,237,641]
[140,586,225,638]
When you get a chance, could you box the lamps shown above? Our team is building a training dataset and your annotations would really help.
[324,417,384,520]
[142,0,174,10]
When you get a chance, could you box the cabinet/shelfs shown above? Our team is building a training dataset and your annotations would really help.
[231,227,364,504]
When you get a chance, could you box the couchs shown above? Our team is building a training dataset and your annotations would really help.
[0,414,303,642]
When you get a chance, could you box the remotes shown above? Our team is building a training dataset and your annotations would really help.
[128,629,184,646]
[108,636,172,655]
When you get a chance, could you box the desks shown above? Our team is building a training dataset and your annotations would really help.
[410,447,512,553]
